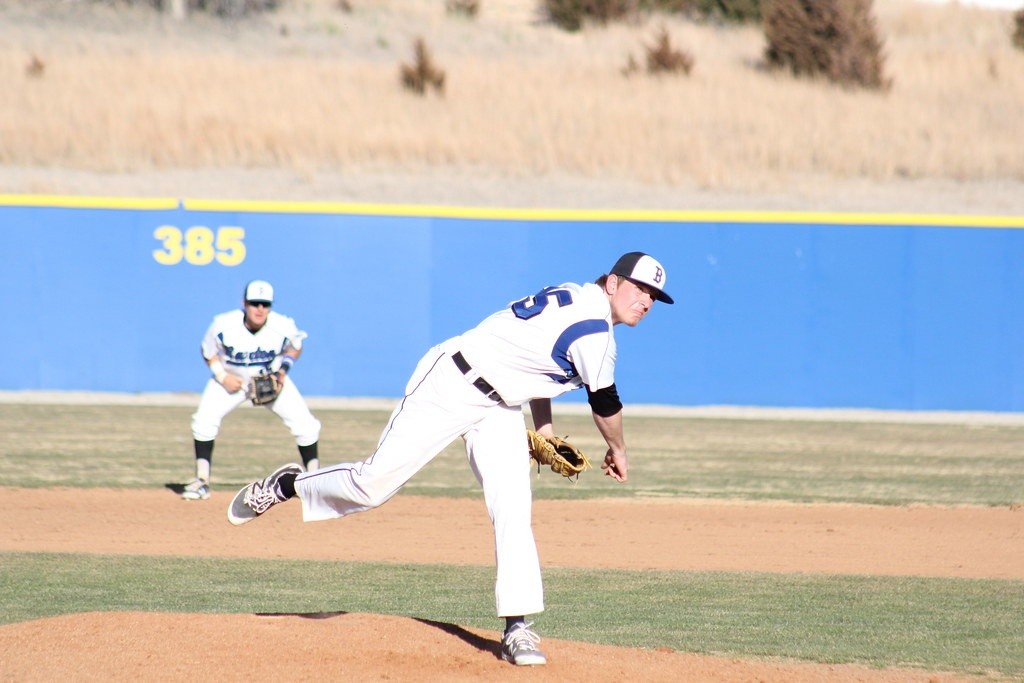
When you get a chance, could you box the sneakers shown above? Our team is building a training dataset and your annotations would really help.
[181,478,211,500]
[501,621,546,666]
[227,463,303,526]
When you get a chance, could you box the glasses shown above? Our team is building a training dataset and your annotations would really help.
[249,300,271,307]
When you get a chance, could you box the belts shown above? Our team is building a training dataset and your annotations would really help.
[226,360,272,366]
[451,350,502,401]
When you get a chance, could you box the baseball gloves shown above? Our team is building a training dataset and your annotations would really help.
[245,369,282,407]
[526,427,588,478]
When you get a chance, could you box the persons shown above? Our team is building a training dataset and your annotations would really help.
[180,282,320,500]
[226,251,674,665]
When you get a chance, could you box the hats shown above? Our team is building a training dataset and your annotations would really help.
[609,252,675,304]
[244,280,274,302]
